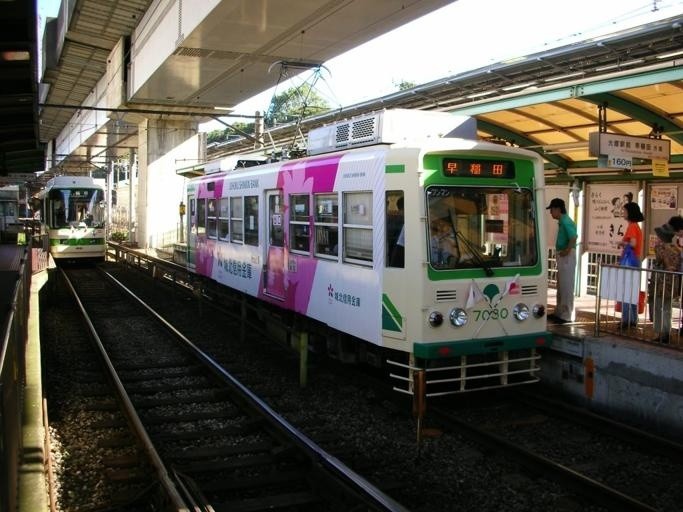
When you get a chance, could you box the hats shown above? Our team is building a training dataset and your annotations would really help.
[654,224,673,243]
[546,198,564,209]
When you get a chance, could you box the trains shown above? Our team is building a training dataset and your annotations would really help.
[28,173,109,265]
[183,106,554,409]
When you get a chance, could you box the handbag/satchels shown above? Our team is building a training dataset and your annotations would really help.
[620,245,641,266]
[615,291,645,314]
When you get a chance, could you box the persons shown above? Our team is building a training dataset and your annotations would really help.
[391,196,404,269]
[649,224,676,344]
[616,202,645,330]
[667,216,682,335]
[545,198,577,324]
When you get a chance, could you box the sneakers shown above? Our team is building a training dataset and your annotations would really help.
[654,336,670,343]
[548,314,565,324]
[616,321,632,328]
